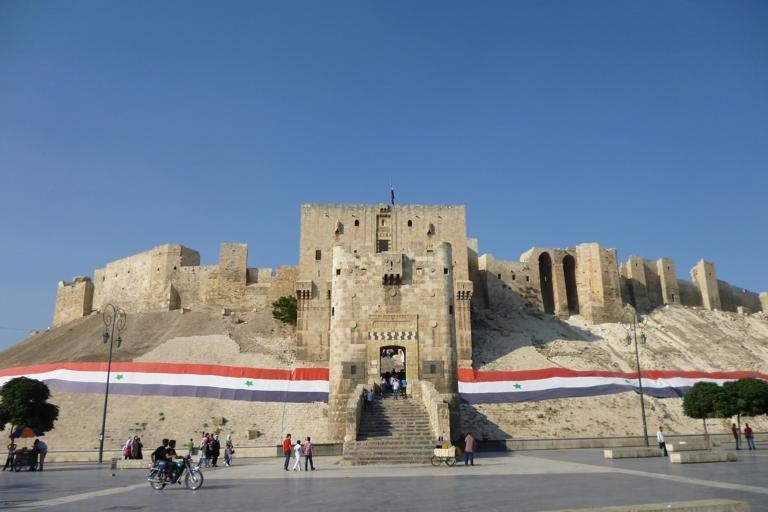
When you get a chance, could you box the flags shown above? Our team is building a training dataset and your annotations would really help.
[390,184,396,206]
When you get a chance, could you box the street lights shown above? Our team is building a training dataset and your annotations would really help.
[99,303,126,464]
[621,309,648,447]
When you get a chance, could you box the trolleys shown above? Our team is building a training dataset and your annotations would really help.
[430,448,459,467]
[14,447,38,472]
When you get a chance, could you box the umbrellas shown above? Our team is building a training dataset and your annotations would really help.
[9,424,45,449]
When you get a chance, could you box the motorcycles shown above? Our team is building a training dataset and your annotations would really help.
[148,454,204,490]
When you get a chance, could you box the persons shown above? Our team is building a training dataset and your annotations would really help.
[3,442,16,470]
[363,349,407,413]
[123,431,234,481]
[731,423,740,449]
[464,431,475,465]
[302,436,315,471]
[744,423,756,449]
[656,426,668,456]
[34,438,48,471]
[292,439,303,470]
[282,433,292,470]
